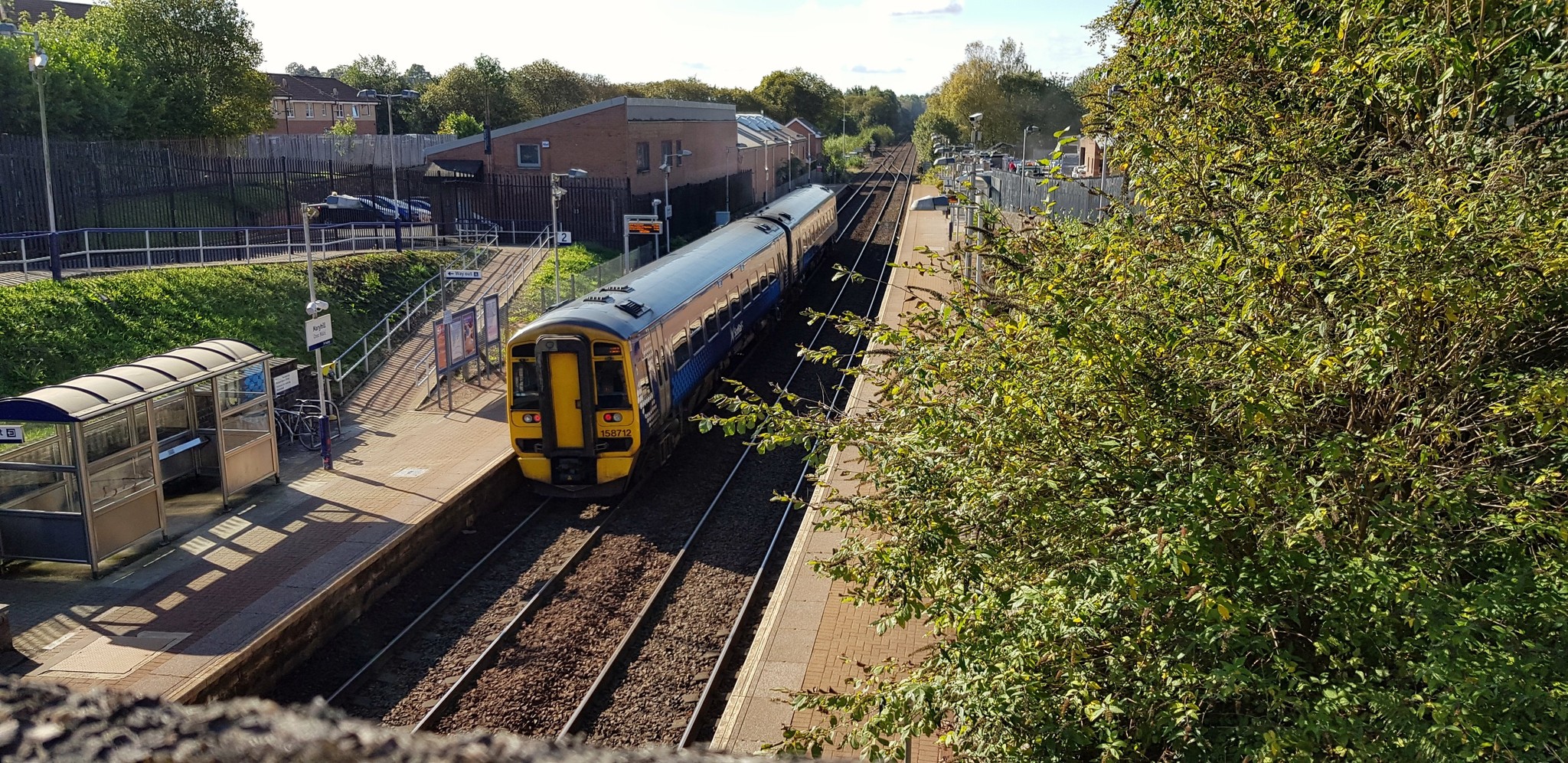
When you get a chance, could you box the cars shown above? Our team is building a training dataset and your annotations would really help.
[314,198,403,228]
[353,193,432,226]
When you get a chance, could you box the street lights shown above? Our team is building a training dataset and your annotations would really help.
[787,134,804,193]
[765,137,785,206]
[930,131,976,285]
[807,132,822,183]
[357,89,420,252]
[661,149,695,253]
[909,194,984,308]
[550,169,588,305]
[1019,125,1039,214]
[726,143,748,211]
[0,22,61,279]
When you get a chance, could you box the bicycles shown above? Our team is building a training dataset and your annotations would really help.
[271,394,322,451]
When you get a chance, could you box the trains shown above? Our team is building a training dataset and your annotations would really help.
[506,184,841,500]
